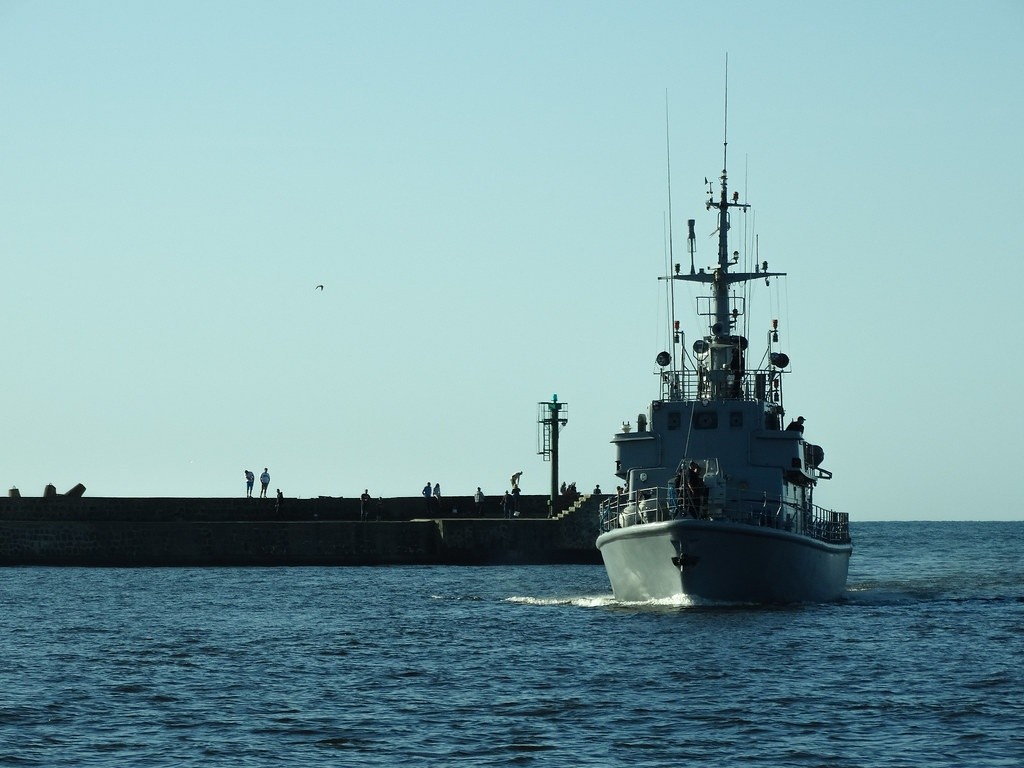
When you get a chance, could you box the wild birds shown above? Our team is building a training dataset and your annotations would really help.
[315,285,323,291]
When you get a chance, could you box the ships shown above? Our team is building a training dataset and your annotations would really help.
[595,47,851,607]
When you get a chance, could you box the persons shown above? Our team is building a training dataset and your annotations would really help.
[275,489,285,521]
[433,483,442,505]
[360,489,370,521]
[259,468,270,498]
[783,415,806,435]
[561,482,581,497]
[245,470,254,498]
[593,485,602,494]
[376,497,384,521]
[510,471,523,488]
[669,461,709,521]
[512,484,520,512]
[422,482,432,515]
[499,490,514,519]
[474,487,487,514]
[617,483,629,493]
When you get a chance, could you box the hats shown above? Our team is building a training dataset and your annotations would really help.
[799,416,806,420]
